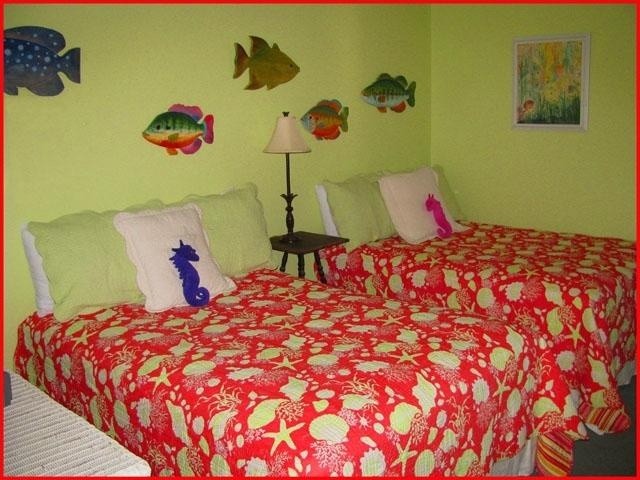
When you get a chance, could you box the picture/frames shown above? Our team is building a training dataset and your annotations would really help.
[511,32,591,134]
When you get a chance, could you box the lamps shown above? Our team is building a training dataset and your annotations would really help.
[263,111,312,244]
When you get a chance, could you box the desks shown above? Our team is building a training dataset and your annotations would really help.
[3,366,152,479]
[270,232,349,285]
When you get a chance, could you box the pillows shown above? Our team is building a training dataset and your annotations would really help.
[429,164,466,223]
[28,210,146,323]
[113,203,238,314]
[314,185,339,239]
[162,182,280,279]
[377,166,471,245]
[324,173,397,254]
[21,224,56,318]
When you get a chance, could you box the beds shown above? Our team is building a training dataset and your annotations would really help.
[313,221,636,407]
[18,269,546,478]
